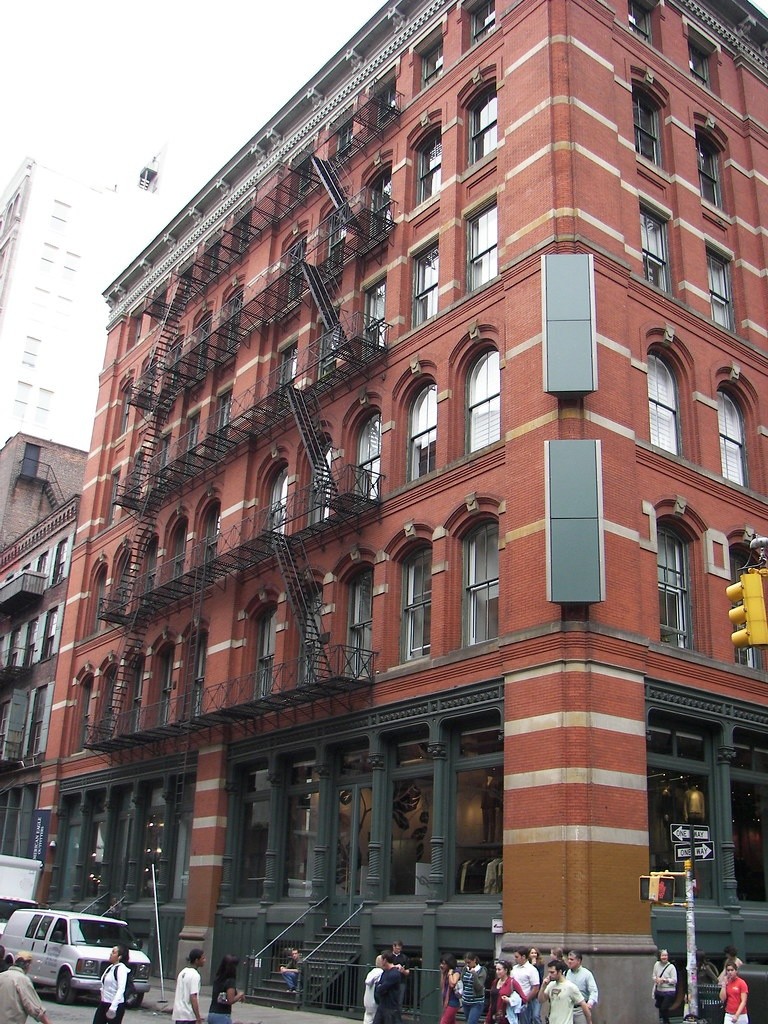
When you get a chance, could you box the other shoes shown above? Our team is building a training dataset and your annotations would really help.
[286,987,304,993]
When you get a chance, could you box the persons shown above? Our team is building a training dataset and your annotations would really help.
[719,963,749,1024]
[0,950,52,1024]
[172,949,206,1024]
[93,945,131,1024]
[562,949,598,1024]
[373,950,403,1024]
[510,945,545,1024]
[280,948,303,993]
[363,955,384,1024]
[391,940,410,1015]
[0,945,12,973]
[439,952,463,1024]
[483,960,527,1024]
[716,944,744,985]
[550,947,569,974]
[477,776,501,844]
[537,960,593,1024]
[461,951,487,1024]
[207,953,245,1024]
[684,785,704,825]
[652,950,677,1024]
[661,785,683,852]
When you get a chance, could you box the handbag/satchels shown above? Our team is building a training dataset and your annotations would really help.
[454,980,464,998]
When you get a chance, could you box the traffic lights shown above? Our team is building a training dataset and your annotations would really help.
[639,876,675,905]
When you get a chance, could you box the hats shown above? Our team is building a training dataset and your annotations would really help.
[14,951,34,965]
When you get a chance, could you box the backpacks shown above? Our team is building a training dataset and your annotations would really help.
[102,964,137,1003]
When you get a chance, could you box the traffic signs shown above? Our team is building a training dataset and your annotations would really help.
[674,841,716,863]
[670,824,710,843]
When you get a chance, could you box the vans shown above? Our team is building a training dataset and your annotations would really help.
[0,909,151,1010]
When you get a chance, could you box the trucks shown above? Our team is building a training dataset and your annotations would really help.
[0,855,44,937]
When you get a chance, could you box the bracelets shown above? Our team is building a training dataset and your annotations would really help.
[447,974,453,978]
[663,979,665,983]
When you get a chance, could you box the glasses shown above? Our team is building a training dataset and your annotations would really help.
[493,961,505,966]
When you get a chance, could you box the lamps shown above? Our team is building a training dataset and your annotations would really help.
[49,840,56,848]
[662,786,671,796]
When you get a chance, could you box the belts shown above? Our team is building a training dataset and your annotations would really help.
[574,1004,581,1008]
